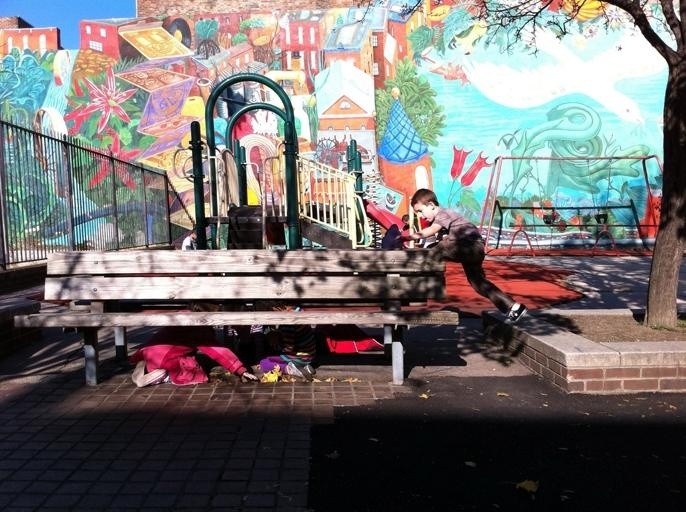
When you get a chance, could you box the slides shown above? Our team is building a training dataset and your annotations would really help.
[363,199,422,248]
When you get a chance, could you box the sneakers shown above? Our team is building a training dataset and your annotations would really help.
[287,362,312,382]
[504,304,528,324]
[137,369,167,388]
[303,364,315,374]
[132,360,145,383]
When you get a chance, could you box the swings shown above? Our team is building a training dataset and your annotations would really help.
[587,158,612,223]
[535,159,564,223]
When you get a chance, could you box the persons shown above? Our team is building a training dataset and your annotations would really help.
[396,189,529,325]
[182,229,197,250]
[381,215,419,249]
[128,299,317,388]
